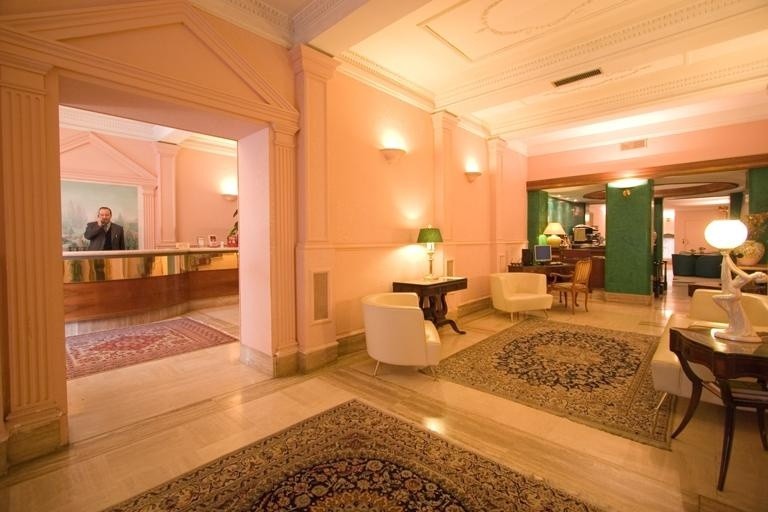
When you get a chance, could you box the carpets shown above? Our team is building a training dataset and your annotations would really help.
[62,317,239,381]
[417,318,678,451]
[102,398,601,512]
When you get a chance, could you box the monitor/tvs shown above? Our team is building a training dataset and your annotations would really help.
[534,245,551,262]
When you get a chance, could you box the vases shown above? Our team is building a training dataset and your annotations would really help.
[728,240,768,266]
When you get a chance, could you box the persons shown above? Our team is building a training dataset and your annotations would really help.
[83,208,125,249]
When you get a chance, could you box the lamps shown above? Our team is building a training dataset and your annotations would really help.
[544,223,566,248]
[221,183,237,202]
[416,225,444,280]
[465,164,481,183]
[704,219,768,343]
[381,138,407,166]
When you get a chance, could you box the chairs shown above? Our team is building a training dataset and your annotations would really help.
[650,288,768,413]
[490,272,553,321]
[362,293,441,376]
[550,260,592,315]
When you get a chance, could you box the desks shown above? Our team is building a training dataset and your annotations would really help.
[670,328,768,492]
[393,277,467,334]
[508,263,579,307]
